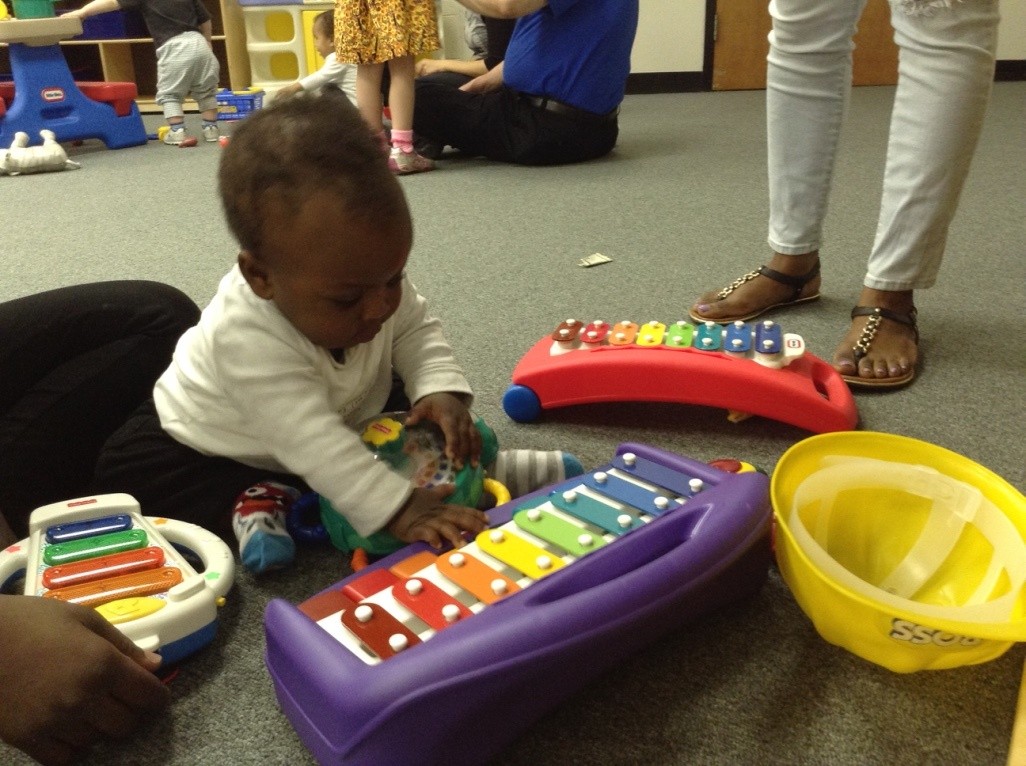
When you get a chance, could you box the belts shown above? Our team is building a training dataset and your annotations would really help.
[517,91,620,125]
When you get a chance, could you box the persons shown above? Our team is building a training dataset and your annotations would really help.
[60,0,220,145]
[333,0,440,176]
[270,7,360,125]
[92,96,480,573]
[1,279,245,766]
[407,0,638,166]
[692,1,1000,391]
[412,9,518,86]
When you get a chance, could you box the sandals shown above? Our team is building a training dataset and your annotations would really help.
[832,295,921,389]
[688,256,824,325]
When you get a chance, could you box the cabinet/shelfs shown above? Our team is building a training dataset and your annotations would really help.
[0,0,248,114]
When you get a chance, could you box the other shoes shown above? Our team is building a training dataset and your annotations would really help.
[407,138,455,159]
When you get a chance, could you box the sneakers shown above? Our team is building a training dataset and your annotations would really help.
[200,125,220,142]
[161,128,188,145]
[380,143,438,175]
